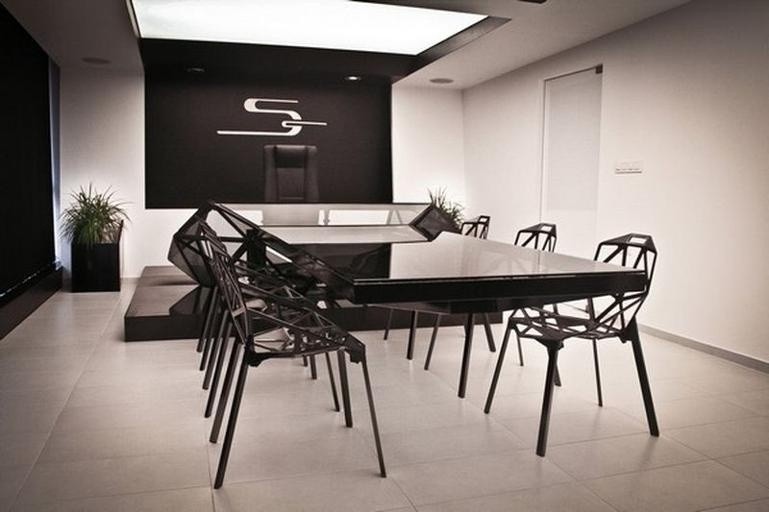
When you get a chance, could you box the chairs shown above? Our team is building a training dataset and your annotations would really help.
[192,221,389,490]
[384,215,660,458]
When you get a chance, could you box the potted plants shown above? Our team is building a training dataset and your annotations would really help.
[57,182,135,293]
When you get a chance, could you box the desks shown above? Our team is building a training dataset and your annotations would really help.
[246,226,646,316]
